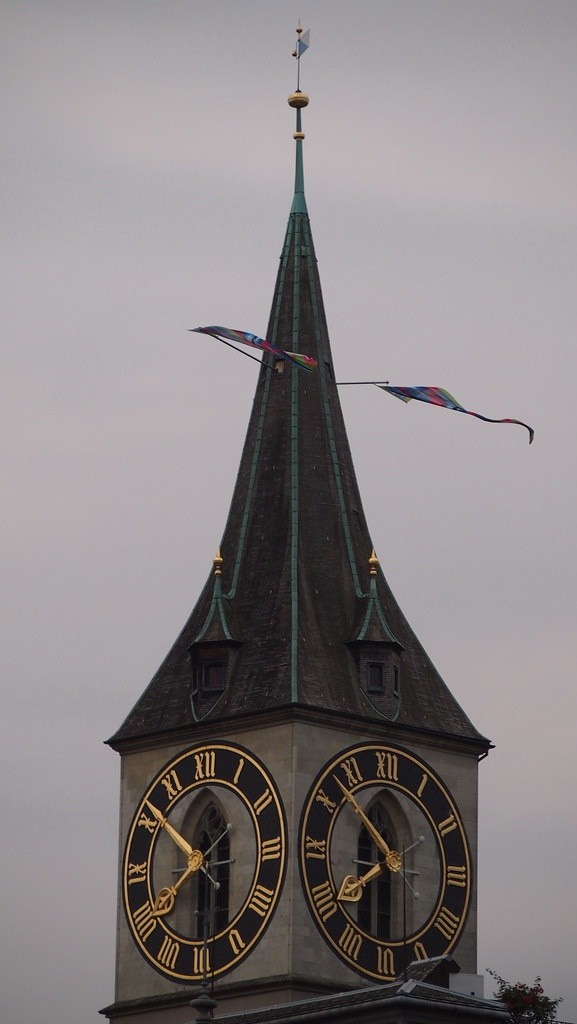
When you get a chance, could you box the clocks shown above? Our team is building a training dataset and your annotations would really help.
[299,740,473,986]
[122,740,288,986]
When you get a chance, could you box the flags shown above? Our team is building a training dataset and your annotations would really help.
[379,385,533,445]
[191,325,317,374]
